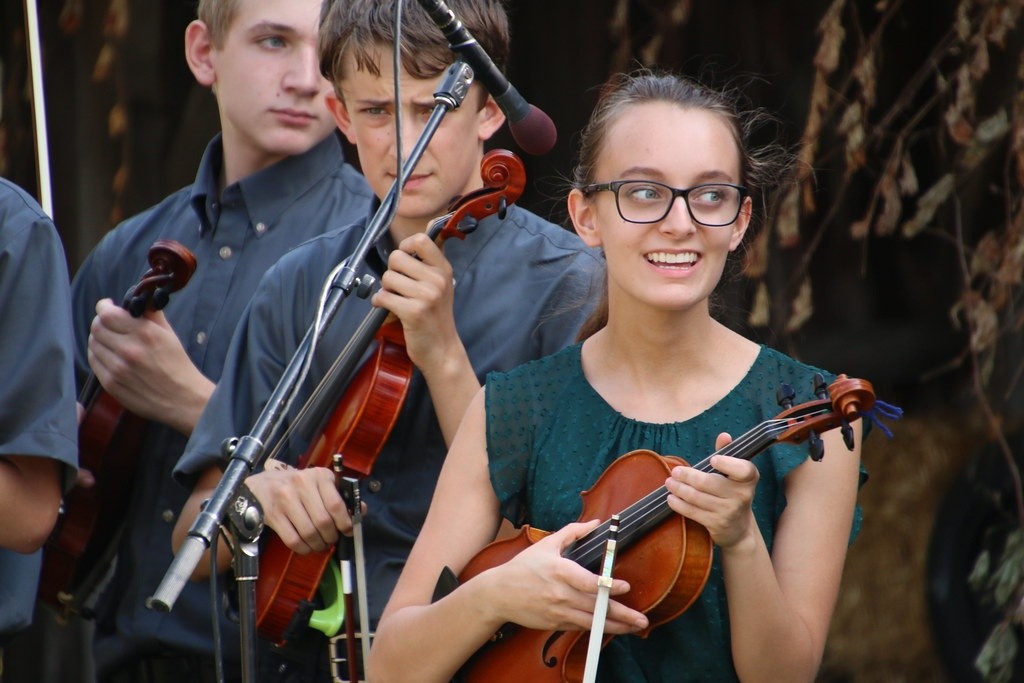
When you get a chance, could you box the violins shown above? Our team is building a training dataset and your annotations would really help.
[249,146,528,651]
[452,369,877,683]
[39,237,201,608]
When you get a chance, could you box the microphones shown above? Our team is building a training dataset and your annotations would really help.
[417,0,558,155]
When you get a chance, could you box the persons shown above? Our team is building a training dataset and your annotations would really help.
[171,1,612,683]
[0,175,82,683]
[361,69,877,682]
[70,3,388,683]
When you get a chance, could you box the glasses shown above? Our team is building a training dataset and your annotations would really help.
[582,180,747,227]
